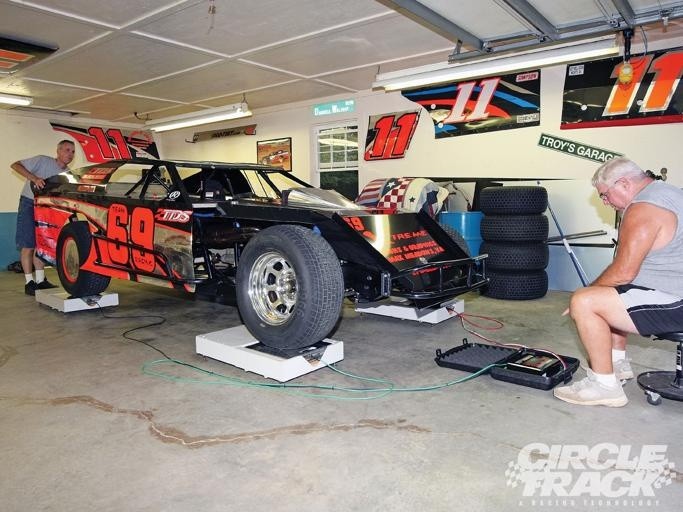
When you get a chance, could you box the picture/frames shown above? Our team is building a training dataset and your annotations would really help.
[256,138,292,174]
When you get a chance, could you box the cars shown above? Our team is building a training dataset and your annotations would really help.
[261,150,288,164]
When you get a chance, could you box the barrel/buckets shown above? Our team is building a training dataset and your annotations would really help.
[439,211,485,267]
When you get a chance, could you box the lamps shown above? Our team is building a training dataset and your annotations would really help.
[144,93,252,132]
[371,33,619,93]
[1,92,34,109]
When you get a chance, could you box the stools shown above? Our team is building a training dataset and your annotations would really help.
[637,330,683,406]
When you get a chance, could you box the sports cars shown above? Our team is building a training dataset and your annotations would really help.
[30,157,490,350]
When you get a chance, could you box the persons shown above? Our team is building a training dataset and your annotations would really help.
[8,140,77,296]
[553,156,683,408]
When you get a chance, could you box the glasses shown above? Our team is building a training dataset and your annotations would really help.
[598,177,624,199]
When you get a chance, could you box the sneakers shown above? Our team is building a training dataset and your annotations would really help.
[25,276,58,296]
[552,357,635,407]
